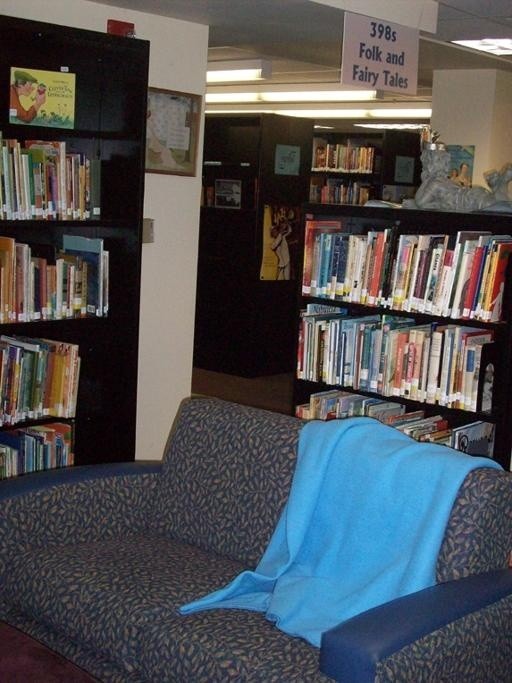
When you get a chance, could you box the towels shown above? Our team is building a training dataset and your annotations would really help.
[176,415,508,649]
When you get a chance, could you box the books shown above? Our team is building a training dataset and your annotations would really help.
[296,390,495,460]
[299,214,511,323]
[310,142,375,205]
[0,130,110,481]
[296,303,494,413]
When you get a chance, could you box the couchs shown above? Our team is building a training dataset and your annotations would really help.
[1,389,510,680]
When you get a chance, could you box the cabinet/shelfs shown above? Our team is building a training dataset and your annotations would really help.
[313,128,421,206]
[196,113,309,379]
[0,14,150,485]
[297,220,512,464]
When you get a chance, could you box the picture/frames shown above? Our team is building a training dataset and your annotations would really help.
[141,84,202,177]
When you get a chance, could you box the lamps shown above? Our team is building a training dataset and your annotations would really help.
[205,54,432,133]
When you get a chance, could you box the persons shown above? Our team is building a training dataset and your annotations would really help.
[9,71,46,124]
